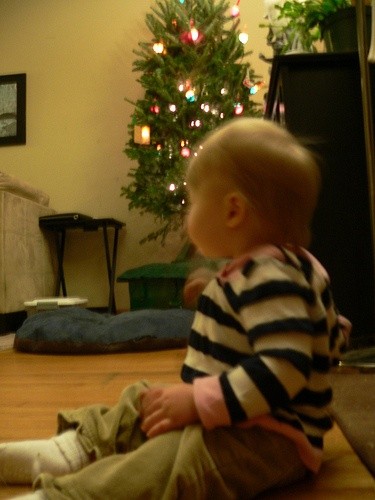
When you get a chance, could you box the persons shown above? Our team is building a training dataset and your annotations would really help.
[0,117,354,500]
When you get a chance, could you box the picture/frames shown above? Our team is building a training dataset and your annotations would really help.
[1,72,26,147]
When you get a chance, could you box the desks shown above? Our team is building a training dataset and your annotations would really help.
[38,212,124,316]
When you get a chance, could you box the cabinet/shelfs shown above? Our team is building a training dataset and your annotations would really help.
[274,50,374,343]
